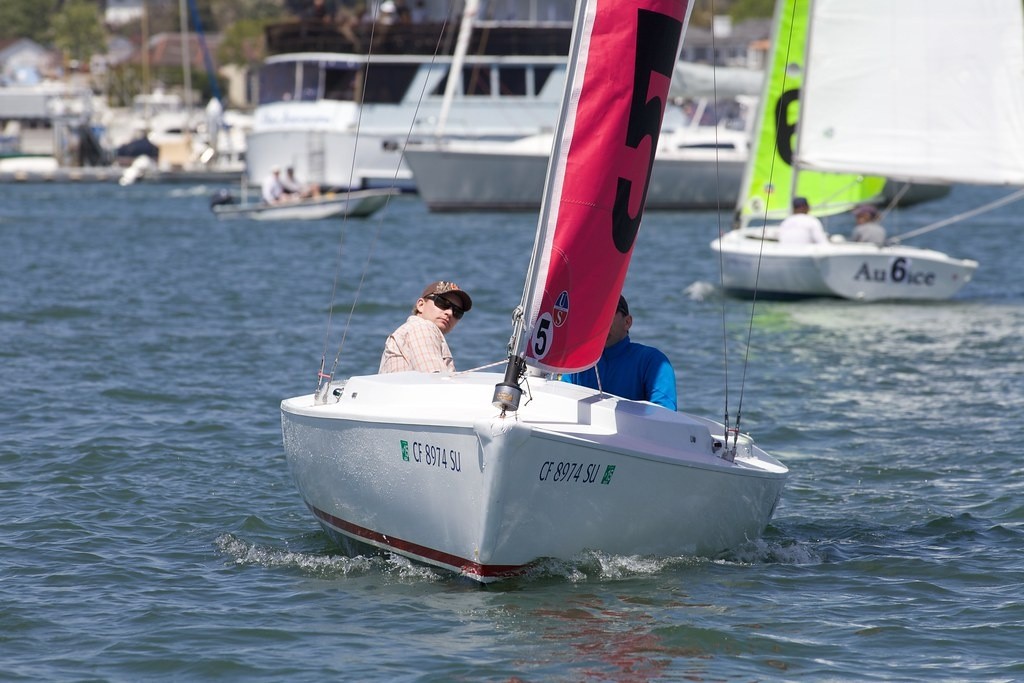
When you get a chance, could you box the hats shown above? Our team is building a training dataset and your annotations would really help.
[618,294,629,315]
[423,281,472,312]
[794,197,807,208]
[854,206,875,217]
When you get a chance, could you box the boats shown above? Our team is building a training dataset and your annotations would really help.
[0,132,250,185]
[209,182,399,217]
[243,53,573,196]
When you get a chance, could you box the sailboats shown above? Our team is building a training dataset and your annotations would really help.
[278,0,788,581]
[712,0,1024,300]
[117,0,252,167]
[400,0,952,212]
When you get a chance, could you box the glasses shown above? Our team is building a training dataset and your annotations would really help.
[425,295,464,319]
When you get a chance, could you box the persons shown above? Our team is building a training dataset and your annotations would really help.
[378,281,472,373]
[559,294,677,412]
[262,167,320,204]
[851,206,886,244]
[778,198,829,244]
